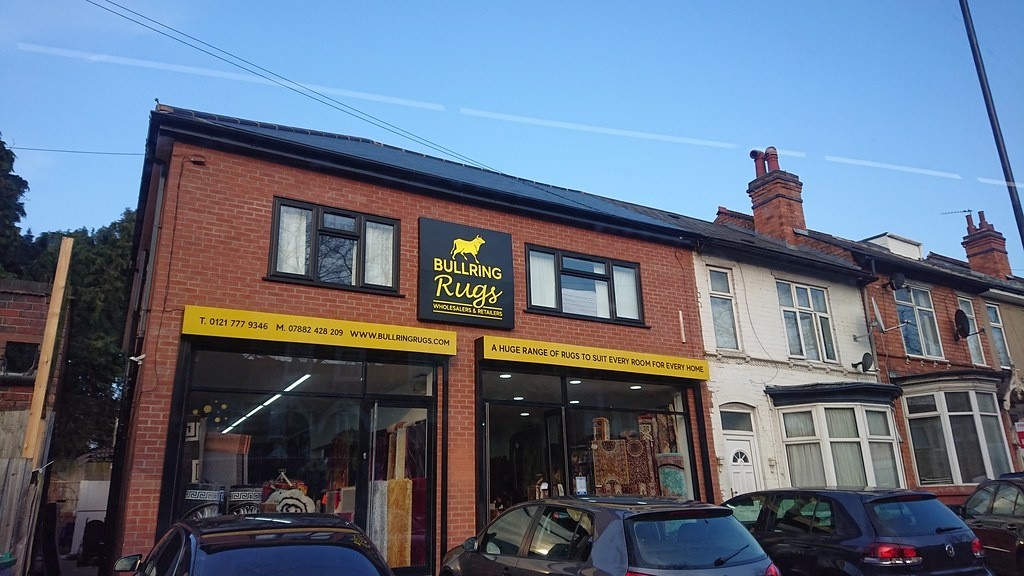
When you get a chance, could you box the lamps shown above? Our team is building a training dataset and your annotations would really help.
[219,372,312,434]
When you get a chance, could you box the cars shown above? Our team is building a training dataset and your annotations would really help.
[439,496,782,576]
[954,470,1024,576]
[113,510,398,576]
[724,487,990,576]
